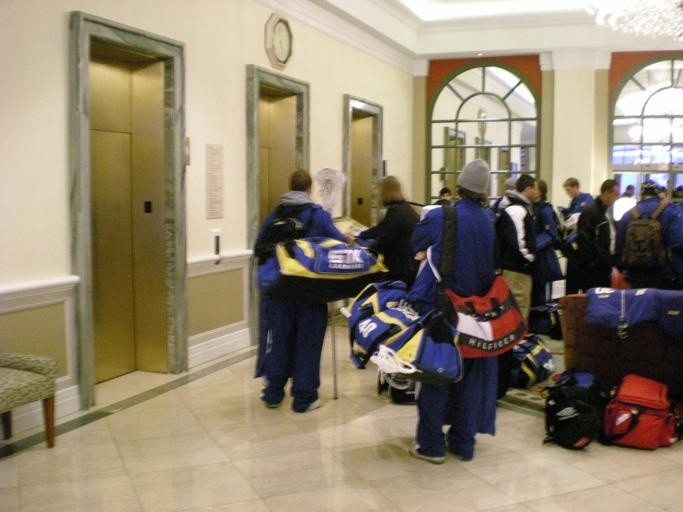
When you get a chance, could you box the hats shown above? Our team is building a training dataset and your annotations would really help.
[456,159,492,195]
[639,179,659,196]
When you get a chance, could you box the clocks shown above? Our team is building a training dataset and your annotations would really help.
[265,13,293,71]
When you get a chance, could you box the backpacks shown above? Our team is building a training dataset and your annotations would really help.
[491,196,529,264]
[620,199,673,278]
[253,201,315,258]
[541,362,604,453]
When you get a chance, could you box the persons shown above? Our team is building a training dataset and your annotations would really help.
[410,158,498,463]
[254,169,354,412]
[353,174,421,287]
[491,176,682,333]
[439,188,452,205]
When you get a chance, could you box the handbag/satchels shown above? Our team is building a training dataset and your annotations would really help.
[253,232,395,307]
[496,331,557,393]
[602,372,678,451]
[345,268,465,387]
[563,208,606,271]
[533,232,567,285]
[432,270,529,361]
[376,365,422,404]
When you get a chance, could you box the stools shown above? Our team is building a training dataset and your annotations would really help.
[0,352,58,448]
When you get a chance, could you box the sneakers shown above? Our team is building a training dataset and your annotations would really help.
[303,398,322,412]
[264,403,281,409]
[407,439,445,465]
